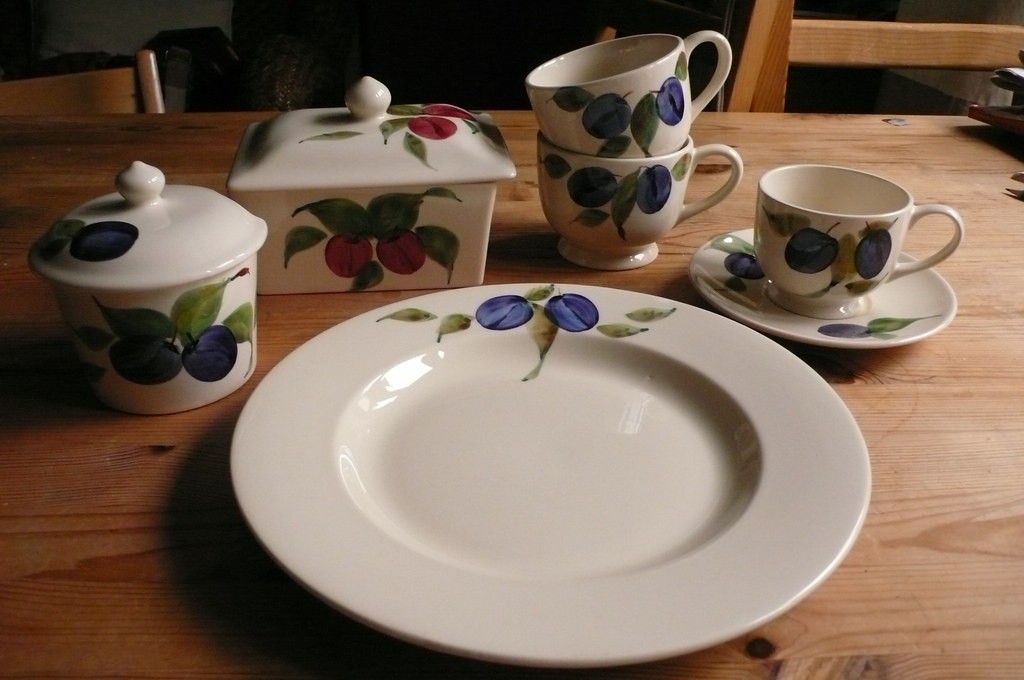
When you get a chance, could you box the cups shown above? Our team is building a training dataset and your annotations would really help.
[753,163,964,318]
[28,159,269,417]
[534,129,742,269]
[525,29,732,161]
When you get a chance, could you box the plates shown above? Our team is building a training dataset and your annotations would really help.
[229,283,872,670]
[687,225,961,349]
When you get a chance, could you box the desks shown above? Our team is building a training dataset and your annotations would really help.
[0,111,1024,679]
